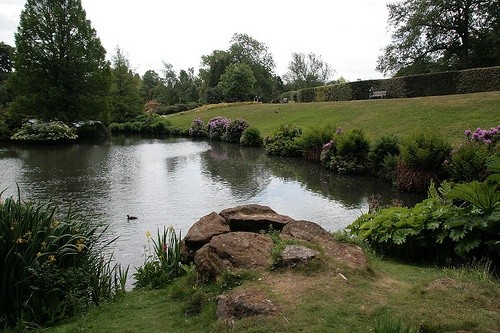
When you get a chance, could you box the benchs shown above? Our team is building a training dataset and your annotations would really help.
[369,91,387,99]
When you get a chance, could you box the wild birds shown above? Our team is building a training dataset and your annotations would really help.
[126,215,138,220]
